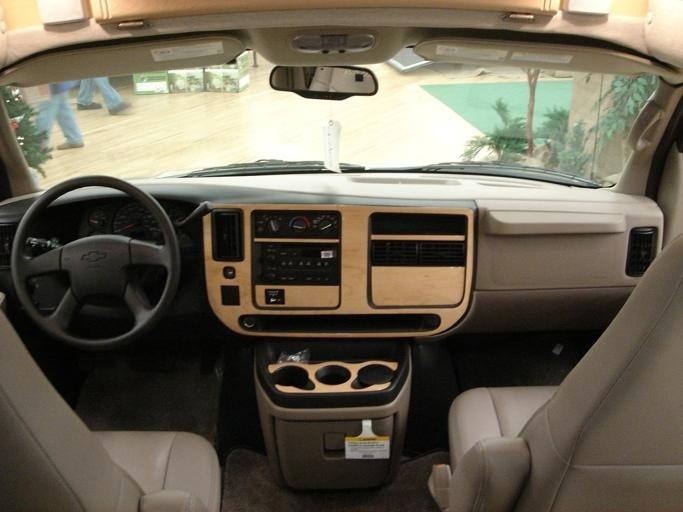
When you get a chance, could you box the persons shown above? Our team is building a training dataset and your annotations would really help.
[35,81,85,154]
[75,78,131,115]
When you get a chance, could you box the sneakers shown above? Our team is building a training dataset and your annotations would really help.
[56,142,84,150]
[77,103,102,111]
[109,101,131,115]
